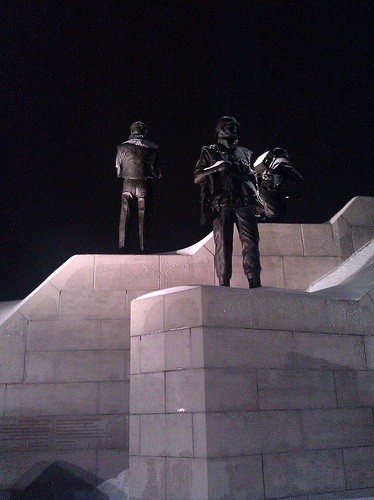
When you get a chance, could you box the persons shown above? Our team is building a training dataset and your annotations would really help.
[195,116,264,288]
[115,121,161,255]
[257,148,305,223]
